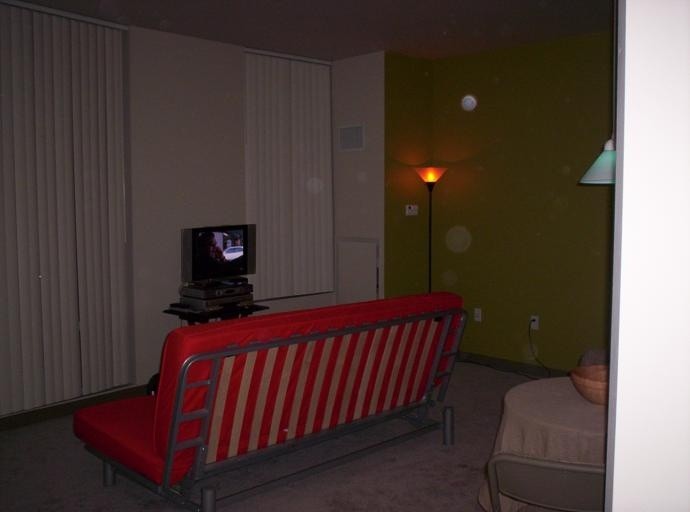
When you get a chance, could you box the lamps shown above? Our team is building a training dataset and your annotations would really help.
[579,0,616,185]
[415,165,449,293]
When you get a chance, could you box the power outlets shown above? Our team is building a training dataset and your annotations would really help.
[530,315,540,330]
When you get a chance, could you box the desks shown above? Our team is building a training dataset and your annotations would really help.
[160,305,270,326]
[477,377,609,512]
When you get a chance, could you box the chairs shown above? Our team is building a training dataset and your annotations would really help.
[487,453,606,512]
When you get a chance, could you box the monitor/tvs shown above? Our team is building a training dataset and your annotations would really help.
[181,224,257,290]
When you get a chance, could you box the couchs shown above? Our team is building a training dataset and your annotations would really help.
[72,289,469,512]
